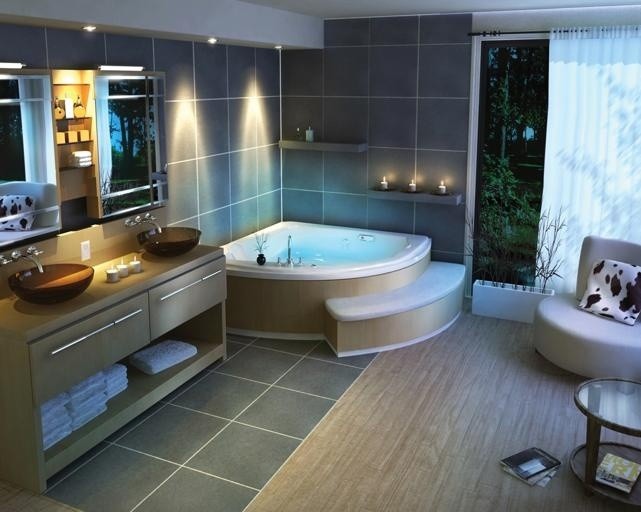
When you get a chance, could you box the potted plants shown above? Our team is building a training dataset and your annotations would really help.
[465,195,568,324]
[255,232,268,265]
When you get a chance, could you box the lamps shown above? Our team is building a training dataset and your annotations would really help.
[94,63,148,72]
[0,61,29,70]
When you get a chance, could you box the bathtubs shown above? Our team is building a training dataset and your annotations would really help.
[221,221,432,281]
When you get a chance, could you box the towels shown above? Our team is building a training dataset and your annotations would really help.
[68,150,93,169]
[37,339,198,446]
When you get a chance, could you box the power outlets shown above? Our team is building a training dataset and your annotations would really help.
[79,240,92,261]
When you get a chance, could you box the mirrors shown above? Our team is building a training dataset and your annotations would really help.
[88,71,169,217]
[0,68,61,251]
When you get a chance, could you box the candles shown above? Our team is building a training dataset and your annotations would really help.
[105,254,142,285]
[377,176,450,195]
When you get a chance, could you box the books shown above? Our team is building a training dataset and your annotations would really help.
[594,453,640,494]
[501,464,559,487]
[535,469,558,489]
[498,446,562,478]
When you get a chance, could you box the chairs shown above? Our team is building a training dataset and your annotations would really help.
[0,179,58,231]
[535,237,641,382]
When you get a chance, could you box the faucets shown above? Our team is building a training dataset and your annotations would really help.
[137,217,163,235]
[11,251,44,275]
[287,234,294,268]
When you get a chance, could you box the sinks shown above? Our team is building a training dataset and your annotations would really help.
[136,226,202,257]
[8,263,95,304]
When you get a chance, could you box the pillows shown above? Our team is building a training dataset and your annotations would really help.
[578,255,641,325]
[0,194,36,231]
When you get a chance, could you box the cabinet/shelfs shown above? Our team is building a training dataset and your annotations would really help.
[51,68,95,171]
[1,255,227,493]
